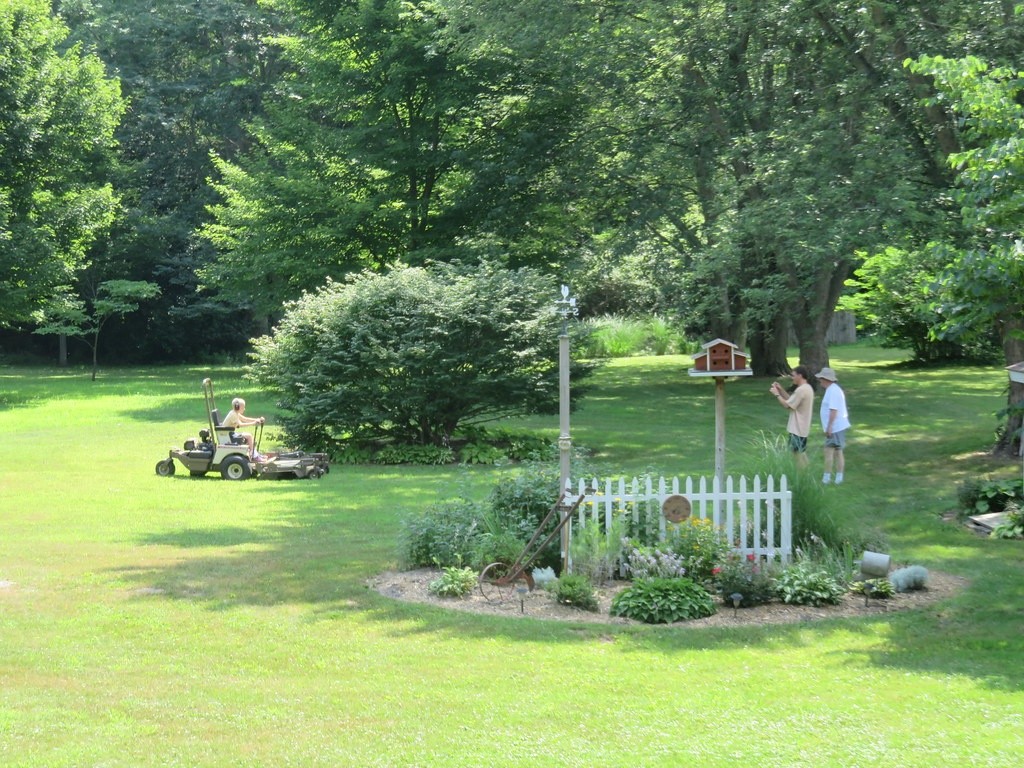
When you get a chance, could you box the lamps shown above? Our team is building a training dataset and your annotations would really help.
[731,592,743,607]
[516,587,527,600]
[863,583,874,595]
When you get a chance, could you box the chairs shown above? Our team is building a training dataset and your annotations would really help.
[212,408,247,445]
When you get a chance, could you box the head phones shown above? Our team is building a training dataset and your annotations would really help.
[235,398,239,410]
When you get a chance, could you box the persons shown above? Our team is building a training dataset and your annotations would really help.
[814,367,851,484]
[769,366,815,467]
[222,398,268,462]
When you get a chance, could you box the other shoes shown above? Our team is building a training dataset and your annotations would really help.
[252,455,268,463]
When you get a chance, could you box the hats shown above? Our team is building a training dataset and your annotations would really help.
[814,367,838,382]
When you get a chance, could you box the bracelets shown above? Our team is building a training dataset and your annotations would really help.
[775,394,780,397]
